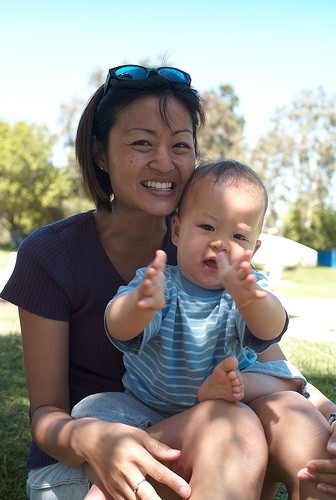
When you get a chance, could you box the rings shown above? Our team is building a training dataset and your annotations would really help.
[134,479,147,493]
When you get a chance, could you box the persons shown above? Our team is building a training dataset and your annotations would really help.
[0,64,335,500]
[66,160,306,500]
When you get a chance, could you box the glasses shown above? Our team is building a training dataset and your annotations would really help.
[103,63,193,96]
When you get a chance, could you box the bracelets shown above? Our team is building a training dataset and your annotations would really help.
[327,412,336,426]
